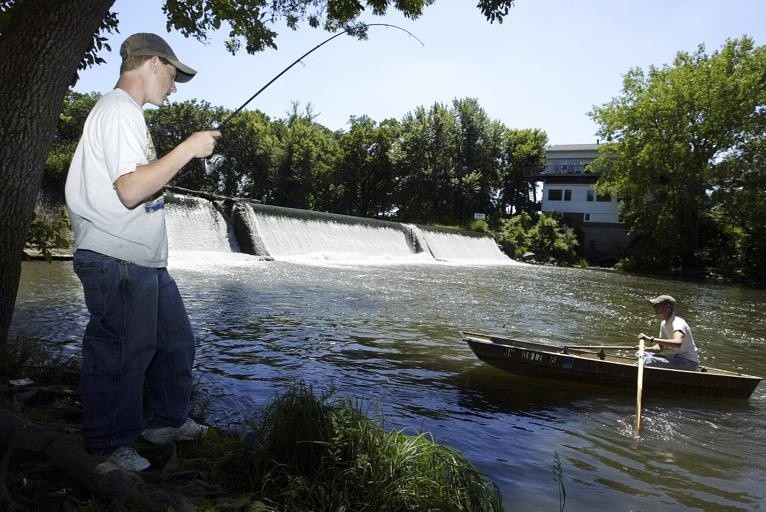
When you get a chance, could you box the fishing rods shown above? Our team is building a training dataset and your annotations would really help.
[217,24,424,132]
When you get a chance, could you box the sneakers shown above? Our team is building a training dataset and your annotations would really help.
[104,443,152,474]
[141,415,212,445]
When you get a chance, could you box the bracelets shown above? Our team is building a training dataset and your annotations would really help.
[651,335,654,343]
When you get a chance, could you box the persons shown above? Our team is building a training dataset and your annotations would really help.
[634,295,699,370]
[63,34,222,473]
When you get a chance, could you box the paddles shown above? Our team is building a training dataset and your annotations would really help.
[637,338,645,434]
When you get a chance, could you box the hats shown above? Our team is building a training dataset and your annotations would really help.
[119,32,199,84]
[647,294,677,307]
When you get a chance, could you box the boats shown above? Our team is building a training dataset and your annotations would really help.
[458,328,762,399]
